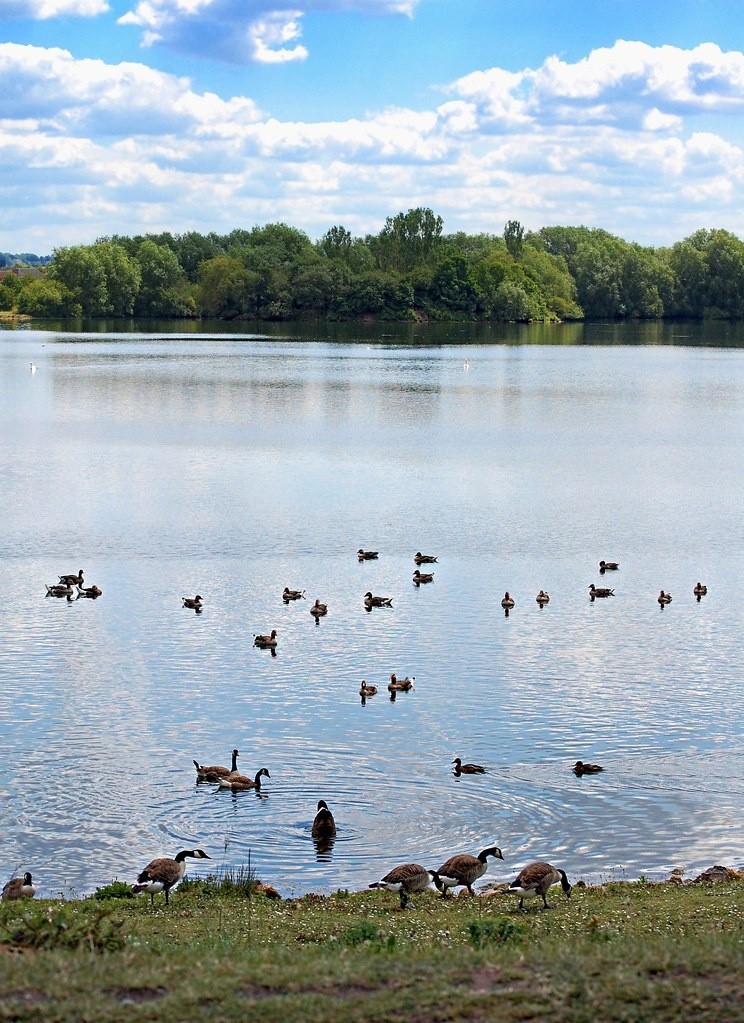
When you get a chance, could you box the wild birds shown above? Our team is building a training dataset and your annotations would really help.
[310,599,328,613]
[432,847,504,898]
[210,768,271,790]
[368,863,446,908]
[451,758,485,772]
[312,799,336,837]
[45,570,102,600]
[388,674,416,691]
[132,849,212,908]
[359,680,378,695]
[501,863,573,911]
[572,761,602,771]
[1,872,36,900]
[501,561,706,610]
[193,749,239,779]
[255,630,278,647]
[283,587,306,599]
[356,548,437,582]
[182,595,204,607]
[363,592,393,608]
